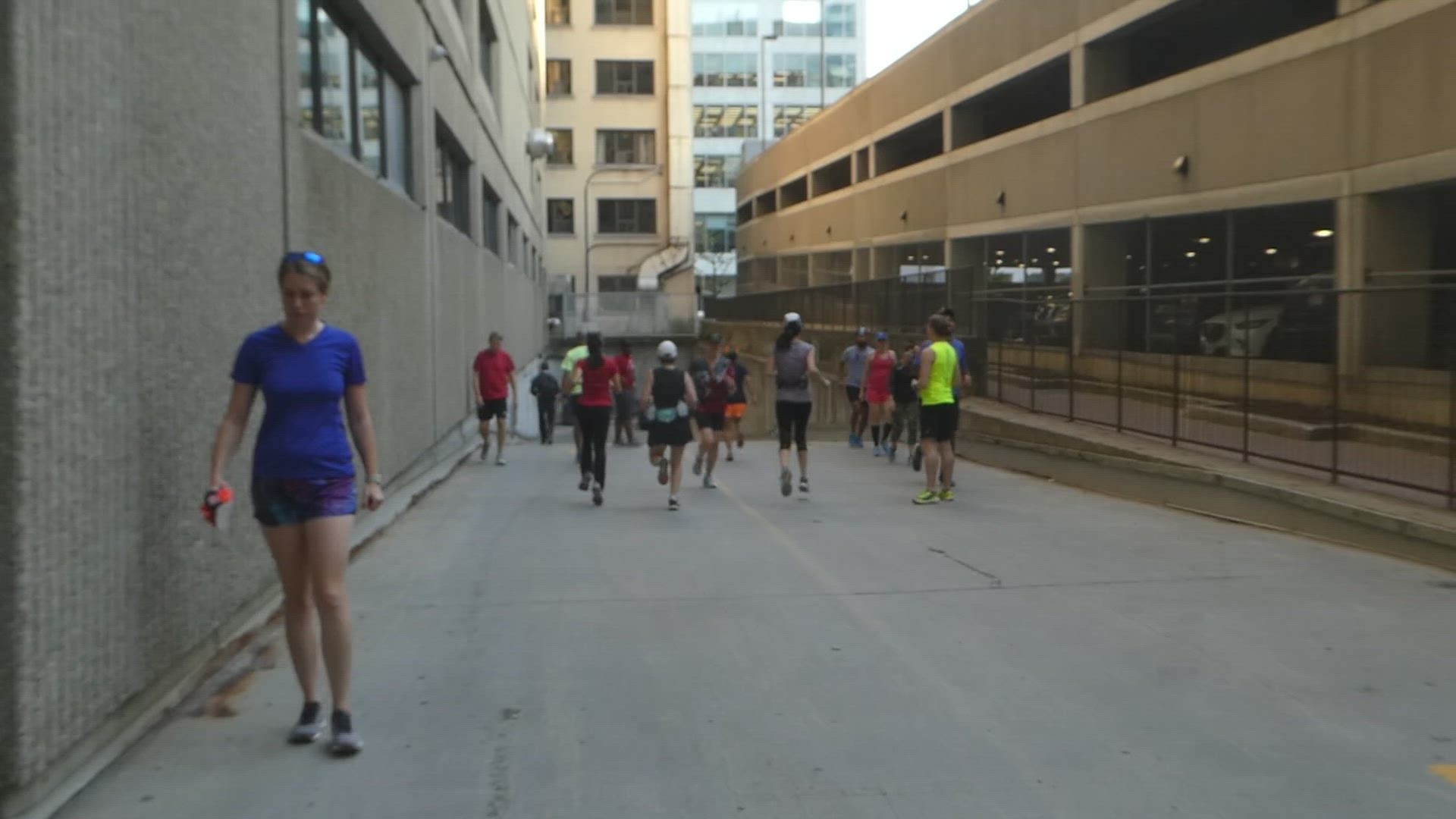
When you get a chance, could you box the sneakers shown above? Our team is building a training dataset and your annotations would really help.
[328,710,362,754]
[873,440,896,462]
[290,700,326,742]
[913,490,941,504]
[938,490,954,501]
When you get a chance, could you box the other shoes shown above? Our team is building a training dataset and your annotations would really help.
[799,479,810,494]
[591,483,604,505]
[497,454,505,464]
[692,456,703,475]
[668,499,679,509]
[579,473,592,490]
[726,455,734,462]
[737,433,744,447]
[481,443,489,460]
[613,436,640,447]
[910,444,922,473]
[780,470,792,496]
[848,432,864,447]
[658,457,669,484]
[939,474,955,486]
[703,480,717,489]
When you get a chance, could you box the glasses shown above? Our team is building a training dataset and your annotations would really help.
[282,250,326,271]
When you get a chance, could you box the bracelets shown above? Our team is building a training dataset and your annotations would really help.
[366,473,382,485]
[474,391,481,396]
[914,382,916,389]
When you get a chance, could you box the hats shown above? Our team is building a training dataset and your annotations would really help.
[878,332,889,341]
[782,312,801,326]
[658,340,678,360]
[856,326,869,337]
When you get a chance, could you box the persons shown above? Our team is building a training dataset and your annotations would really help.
[837,308,971,504]
[561,331,759,510]
[208,250,384,759]
[765,312,830,497]
[472,332,518,466]
[530,362,560,446]
